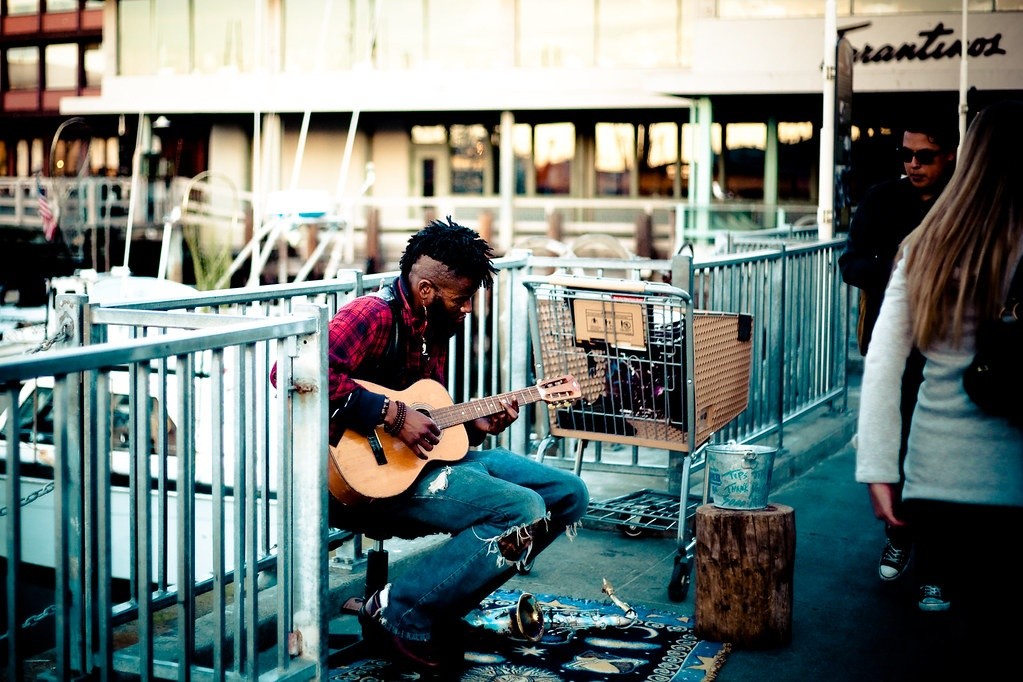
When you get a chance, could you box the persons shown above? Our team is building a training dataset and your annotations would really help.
[854,102,1023,682]
[839,125,959,610]
[270,215,589,672]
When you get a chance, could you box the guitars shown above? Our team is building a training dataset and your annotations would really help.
[327,373,582,510]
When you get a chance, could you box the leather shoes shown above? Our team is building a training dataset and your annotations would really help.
[359,589,463,671]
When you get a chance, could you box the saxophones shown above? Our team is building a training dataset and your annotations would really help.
[463,577,639,643]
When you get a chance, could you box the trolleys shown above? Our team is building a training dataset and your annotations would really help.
[521,275,755,604]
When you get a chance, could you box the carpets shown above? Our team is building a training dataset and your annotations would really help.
[267,586,736,682]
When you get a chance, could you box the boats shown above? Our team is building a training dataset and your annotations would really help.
[0,102,322,615]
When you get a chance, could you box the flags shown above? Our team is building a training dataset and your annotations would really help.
[36,177,56,240]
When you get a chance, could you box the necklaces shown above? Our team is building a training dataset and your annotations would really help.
[422,337,428,355]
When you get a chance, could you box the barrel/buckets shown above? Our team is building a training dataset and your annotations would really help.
[706,440,777,510]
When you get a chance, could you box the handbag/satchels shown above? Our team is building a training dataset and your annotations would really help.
[963,254,1022,418]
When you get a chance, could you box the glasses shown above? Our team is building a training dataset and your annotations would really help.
[896,145,946,165]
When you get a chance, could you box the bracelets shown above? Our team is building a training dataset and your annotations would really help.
[382,397,389,417]
[384,401,406,436]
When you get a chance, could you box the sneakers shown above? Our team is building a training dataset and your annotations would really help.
[917,581,951,612]
[879,538,911,581]
